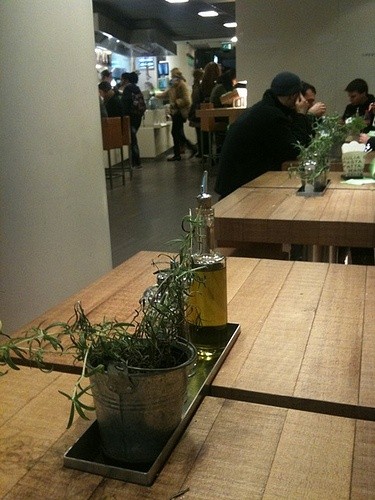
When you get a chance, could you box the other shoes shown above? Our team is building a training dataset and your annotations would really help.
[167,155,181,161]
[189,146,198,159]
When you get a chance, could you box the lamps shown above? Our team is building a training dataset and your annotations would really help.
[197,7,219,18]
[223,19,238,28]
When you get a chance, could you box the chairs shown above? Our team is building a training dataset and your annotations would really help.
[101,115,134,189]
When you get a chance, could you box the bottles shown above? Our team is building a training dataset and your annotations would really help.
[184,194,227,362]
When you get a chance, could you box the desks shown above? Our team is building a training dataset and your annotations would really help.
[0,250,375,414]
[194,107,245,167]
[212,163,375,263]
[137,120,173,158]
[0,359,375,500]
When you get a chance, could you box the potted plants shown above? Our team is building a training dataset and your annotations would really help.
[285,110,368,183]
[0,207,231,442]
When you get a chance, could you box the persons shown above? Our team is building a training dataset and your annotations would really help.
[299,80,327,143]
[189,60,236,173]
[98,69,129,117]
[339,78,375,132]
[359,132,375,152]
[214,72,309,196]
[153,67,197,161]
[123,72,145,169]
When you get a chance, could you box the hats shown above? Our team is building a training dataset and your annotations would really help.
[271,71,303,96]
